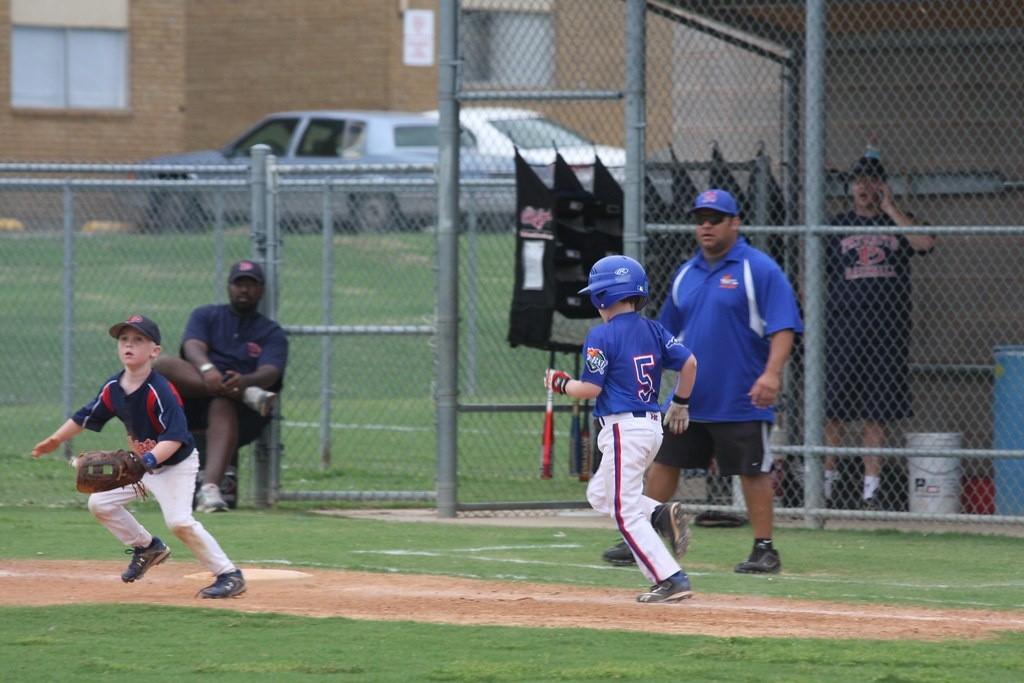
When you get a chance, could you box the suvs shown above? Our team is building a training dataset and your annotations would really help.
[127,110,485,233]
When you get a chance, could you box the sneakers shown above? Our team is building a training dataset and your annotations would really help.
[654,502,692,559]
[636,571,694,602]
[122,536,171,582]
[202,569,247,600]
[242,385,277,417]
[734,546,781,574]
[601,542,634,564]
[195,484,229,513]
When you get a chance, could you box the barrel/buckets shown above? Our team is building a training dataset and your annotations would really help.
[993,345,1024,516]
[904,432,965,514]
[963,479,994,514]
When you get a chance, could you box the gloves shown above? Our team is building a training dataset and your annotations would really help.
[662,394,690,434]
[544,368,571,394]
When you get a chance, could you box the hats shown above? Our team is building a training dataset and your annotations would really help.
[227,260,264,284]
[109,314,161,344]
[686,190,740,215]
[849,158,884,176]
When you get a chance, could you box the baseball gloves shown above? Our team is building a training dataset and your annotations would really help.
[74,446,146,494]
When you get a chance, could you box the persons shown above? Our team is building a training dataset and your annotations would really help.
[30,315,249,599]
[819,154,937,514]
[179,260,289,514]
[599,188,803,574]
[543,255,698,605]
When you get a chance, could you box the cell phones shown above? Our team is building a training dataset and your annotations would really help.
[872,191,884,206]
[222,374,232,383]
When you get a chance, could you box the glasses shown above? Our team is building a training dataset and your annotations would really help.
[694,214,728,225]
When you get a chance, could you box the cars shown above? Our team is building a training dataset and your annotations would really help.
[426,107,626,229]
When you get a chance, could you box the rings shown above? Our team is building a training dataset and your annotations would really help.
[234,387,241,394]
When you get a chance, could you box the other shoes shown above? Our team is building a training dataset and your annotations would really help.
[860,488,886,510]
[824,479,841,507]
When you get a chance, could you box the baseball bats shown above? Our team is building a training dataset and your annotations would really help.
[539,370,557,479]
[578,399,592,481]
[568,396,579,479]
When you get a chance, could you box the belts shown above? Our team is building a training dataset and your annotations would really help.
[601,411,646,426]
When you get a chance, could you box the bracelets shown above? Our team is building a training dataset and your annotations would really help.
[199,362,215,374]
[141,451,157,470]
[673,394,689,404]
